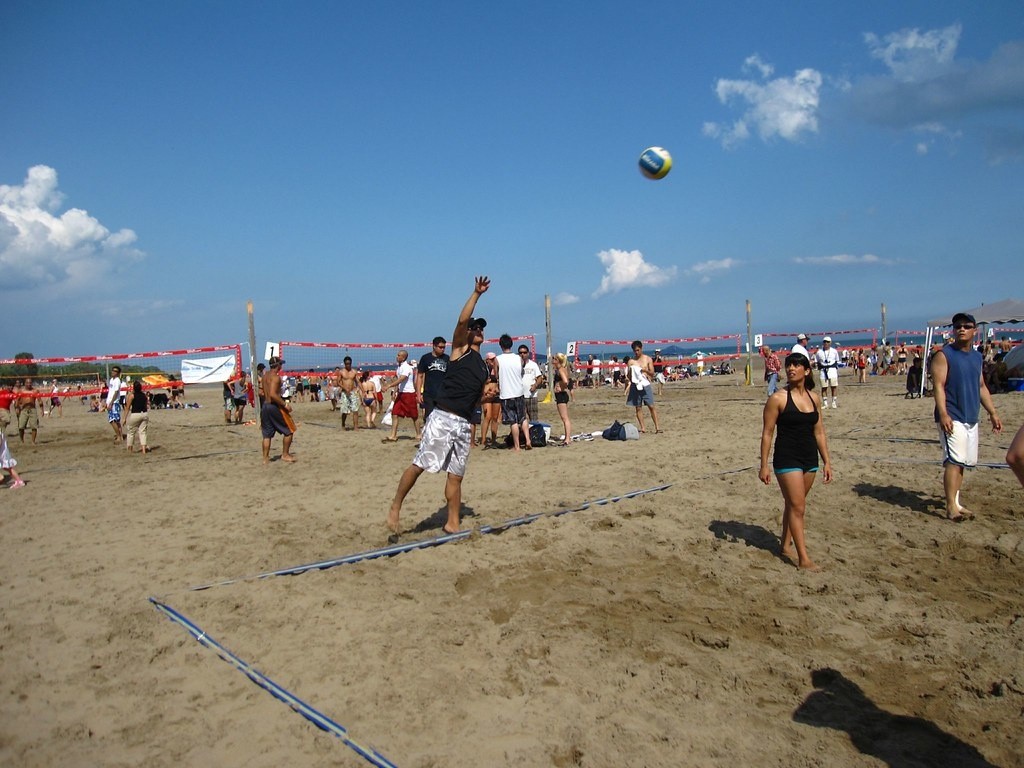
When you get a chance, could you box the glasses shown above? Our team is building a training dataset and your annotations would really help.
[437,346,445,349]
[519,352,527,354]
[467,325,484,331]
[952,324,975,329]
[823,340,830,343]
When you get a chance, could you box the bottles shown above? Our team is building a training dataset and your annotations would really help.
[285,399,289,406]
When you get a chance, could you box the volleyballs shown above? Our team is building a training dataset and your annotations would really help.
[638,147,673,179]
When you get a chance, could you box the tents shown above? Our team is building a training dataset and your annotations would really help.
[920,298,1024,396]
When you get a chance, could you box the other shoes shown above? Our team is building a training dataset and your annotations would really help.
[821,405,828,409]
[10,481,25,488]
[832,403,837,408]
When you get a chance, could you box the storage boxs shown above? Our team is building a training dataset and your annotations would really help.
[528,422,552,443]
[1007,377,1024,391]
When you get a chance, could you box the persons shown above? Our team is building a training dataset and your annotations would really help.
[388,275,491,534]
[758,353,832,568]
[469,333,548,450]
[1006,425,1024,487]
[0,433,25,490]
[573,349,735,388]
[0,349,422,464]
[816,337,840,409]
[973,336,1011,362]
[625,341,662,433]
[883,342,922,376]
[790,334,815,367]
[842,347,871,383]
[932,312,1003,520]
[418,337,450,418]
[907,358,927,395]
[943,338,954,347]
[552,353,571,446]
[762,346,782,396]
[568,377,575,401]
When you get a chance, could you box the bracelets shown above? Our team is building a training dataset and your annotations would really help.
[284,403,287,407]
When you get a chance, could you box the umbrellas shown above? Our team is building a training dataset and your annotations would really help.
[142,374,170,389]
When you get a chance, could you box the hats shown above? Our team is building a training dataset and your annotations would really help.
[485,352,496,359]
[468,317,486,328]
[823,336,831,341]
[797,334,810,340]
[952,313,975,323]
[900,343,904,346]
[269,357,285,366]
[913,358,923,363]
